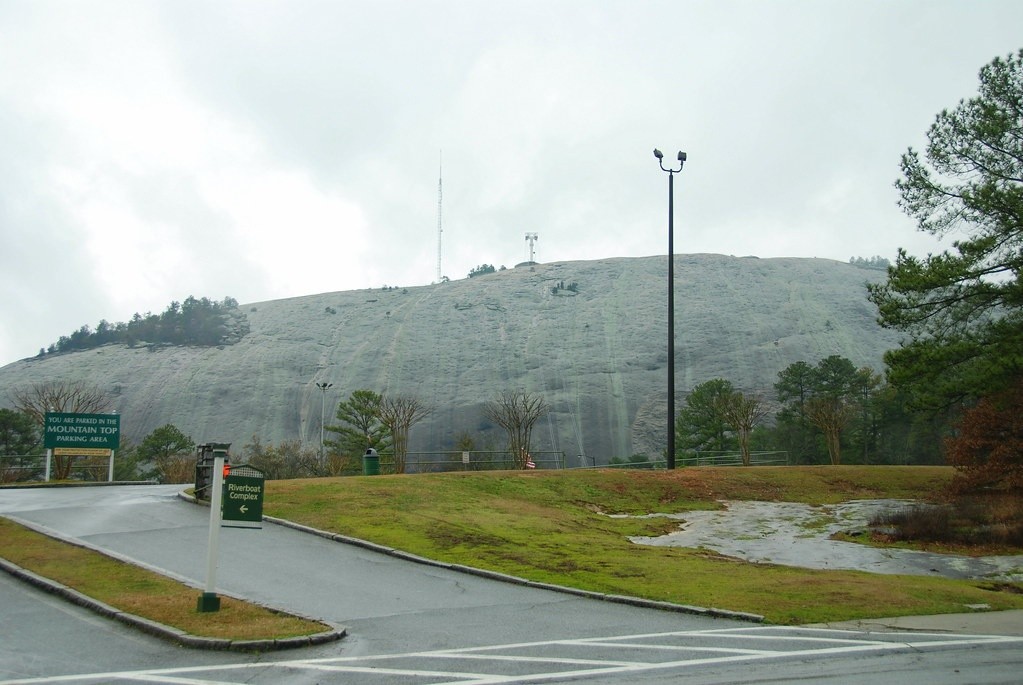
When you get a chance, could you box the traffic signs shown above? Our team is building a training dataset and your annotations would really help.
[221,466,265,529]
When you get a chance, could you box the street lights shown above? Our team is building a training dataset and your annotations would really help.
[316,382,333,466]
[653,146,686,472]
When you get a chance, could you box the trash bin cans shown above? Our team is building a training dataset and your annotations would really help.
[363,448,380,476]
